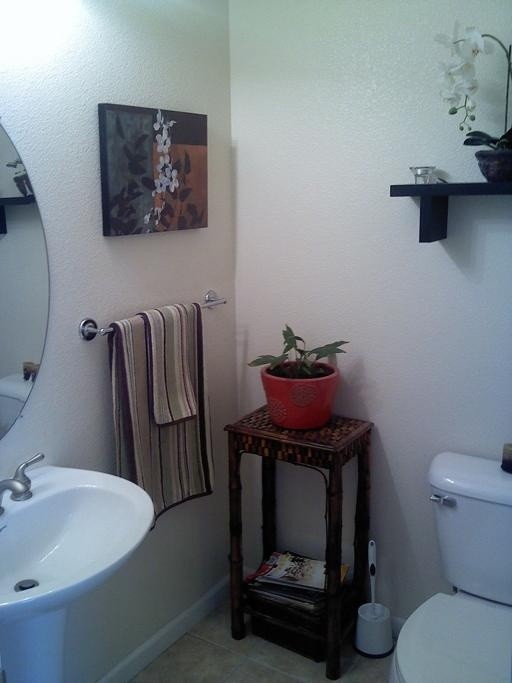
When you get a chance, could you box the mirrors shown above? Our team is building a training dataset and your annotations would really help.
[0,124,51,445]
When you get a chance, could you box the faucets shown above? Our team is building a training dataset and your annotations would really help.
[1,478,32,513]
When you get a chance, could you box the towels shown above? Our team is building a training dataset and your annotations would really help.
[108,300,216,531]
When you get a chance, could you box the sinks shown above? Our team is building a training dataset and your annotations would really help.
[0,468,157,623]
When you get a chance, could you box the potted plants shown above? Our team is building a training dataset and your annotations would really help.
[248,323,350,432]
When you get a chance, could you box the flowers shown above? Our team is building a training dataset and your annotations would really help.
[433,21,512,151]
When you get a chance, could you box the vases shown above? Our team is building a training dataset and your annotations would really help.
[477,150,512,184]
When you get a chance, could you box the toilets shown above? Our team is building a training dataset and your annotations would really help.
[387,452,512,682]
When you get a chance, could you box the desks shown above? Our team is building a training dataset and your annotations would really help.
[223,400,375,682]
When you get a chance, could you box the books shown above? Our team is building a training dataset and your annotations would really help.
[244,550,350,628]
